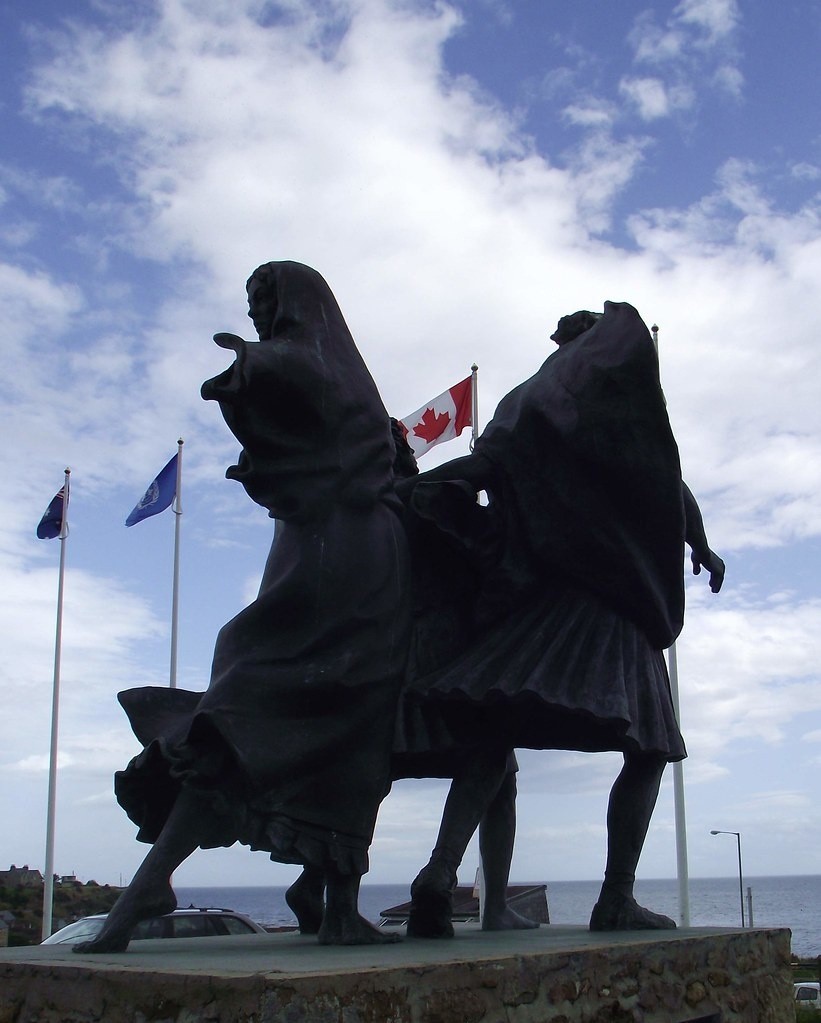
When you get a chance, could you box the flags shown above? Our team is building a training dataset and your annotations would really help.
[124,453,179,526]
[36,485,70,540]
[396,375,471,458]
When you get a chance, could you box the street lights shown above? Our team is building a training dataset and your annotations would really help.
[710,830,745,928]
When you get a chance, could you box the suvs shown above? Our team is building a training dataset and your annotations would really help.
[37,906,268,945]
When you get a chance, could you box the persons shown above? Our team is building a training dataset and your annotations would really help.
[71,260,726,946]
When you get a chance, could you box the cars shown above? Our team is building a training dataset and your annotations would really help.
[793,982,821,1011]
